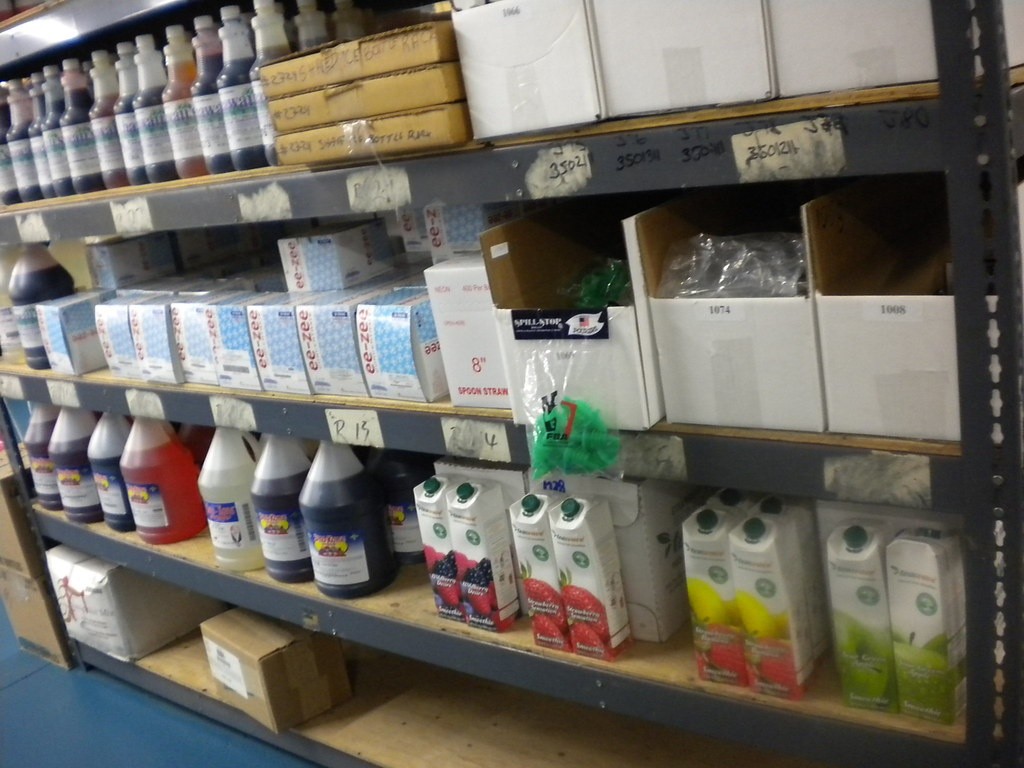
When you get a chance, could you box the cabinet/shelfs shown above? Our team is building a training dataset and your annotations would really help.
[0,0,1024,768]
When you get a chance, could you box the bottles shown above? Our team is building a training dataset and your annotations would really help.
[86,411,137,534]
[7,241,76,371]
[249,435,312,585]
[47,407,104,523]
[198,427,264,573]
[0,0,365,208]
[119,418,213,545]
[299,439,424,598]
[21,402,60,510]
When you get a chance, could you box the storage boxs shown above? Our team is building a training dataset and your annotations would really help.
[45,545,228,664]
[452,0,607,139]
[199,607,352,735]
[36,202,481,404]
[593,0,779,117]
[435,455,964,644]
[266,59,464,131]
[275,101,470,167]
[424,250,512,410]
[636,193,828,433]
[476,199,665,431]
[805,182,1024,442]
[0,441,77,672]
[258,20,457,102]
[768,0,1024,98]
